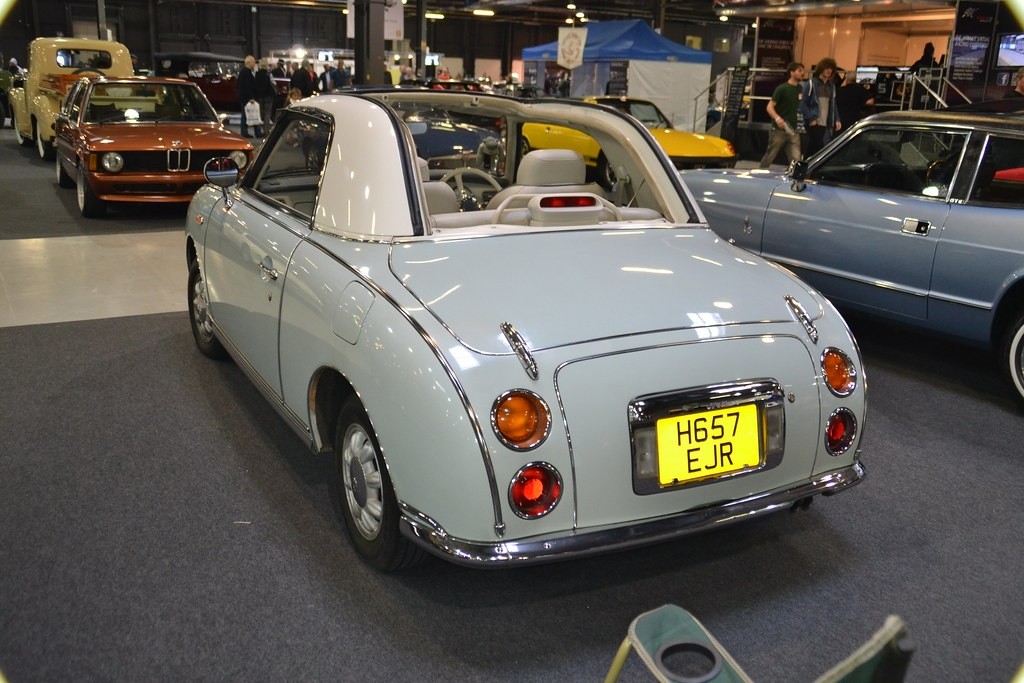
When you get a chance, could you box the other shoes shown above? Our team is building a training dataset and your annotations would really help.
[256,133,265,139]
[241,133,254,138]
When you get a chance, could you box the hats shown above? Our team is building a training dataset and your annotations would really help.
[277,59,285,65]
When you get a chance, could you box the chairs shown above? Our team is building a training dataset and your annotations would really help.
[89,102,119,123]
[486,147,609,212]
[153,103,181,122]
[415,156,462,217]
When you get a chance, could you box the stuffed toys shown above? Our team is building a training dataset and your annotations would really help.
[476,136,505,168]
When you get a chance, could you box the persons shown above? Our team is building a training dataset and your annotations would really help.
[1004,67,1024,98]
[384,64,512,90]
[8,58,24,87]
[236,54,352,139]
[760,42,938,170]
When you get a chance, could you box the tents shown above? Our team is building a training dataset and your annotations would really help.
[522,17,712,132]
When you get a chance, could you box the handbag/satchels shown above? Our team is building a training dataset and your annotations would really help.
[245,101,263,125]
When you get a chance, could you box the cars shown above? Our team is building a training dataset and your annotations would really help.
[9,37,134,159]
[184,93,868,578]
[53,71,258,218]
[296,105,505,170]
[678,111,1024,415]
[523,94,736,170]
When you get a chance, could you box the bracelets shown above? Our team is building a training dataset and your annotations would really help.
[775,117,780,122]
